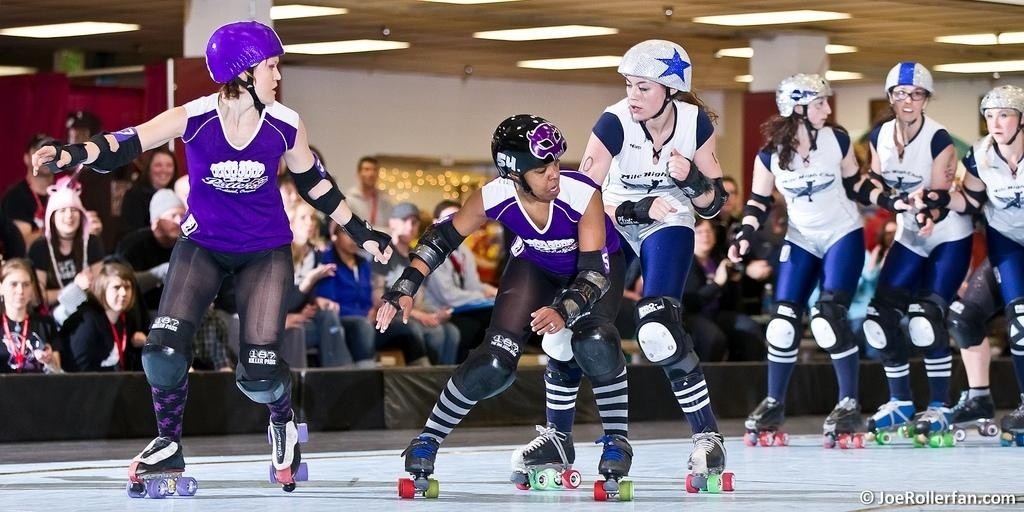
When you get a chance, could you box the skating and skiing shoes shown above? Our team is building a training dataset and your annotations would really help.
[126,437,197,499]
[824,392,1023,450]
[747,397,788,447]
[397,437,437,499]
[685,432,734,493]
[269,412,310,490]
[510,433,579,488]
[592,437,634,501]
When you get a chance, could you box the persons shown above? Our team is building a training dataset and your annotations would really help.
[860,62,976,437]
[715,73,922,438]
[1,113,500,375]
[915,84,1024,431]
[611,176,1021,405]
[30,17,393,484]
[376,115,632,477]
[509,41,728,470]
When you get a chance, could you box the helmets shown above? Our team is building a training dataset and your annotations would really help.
[982,85,1023,116]
[492,113,565,189]
[617,39,693,98]
[775,76,830,117]
[207,22,283,84]
[885,61,934,97]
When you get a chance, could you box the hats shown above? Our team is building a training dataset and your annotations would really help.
[150,189,185,226]
[391,205,419,219]
[43,183,90,238]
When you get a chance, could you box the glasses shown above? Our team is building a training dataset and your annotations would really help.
[893,90,927,103]
